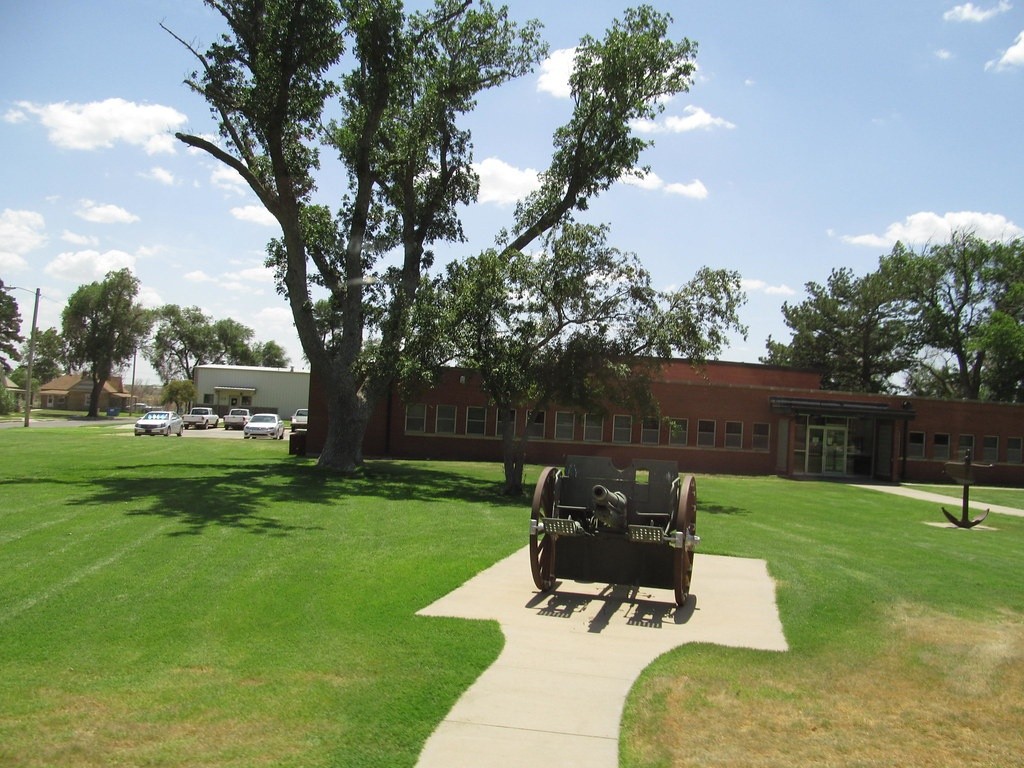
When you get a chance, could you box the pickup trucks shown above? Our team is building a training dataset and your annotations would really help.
[291,409,308,431]
[182,406,220,429]
[223,409,251,431]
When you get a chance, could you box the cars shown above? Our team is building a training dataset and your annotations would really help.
[243,413,285,440]
[135,411,184,438]
